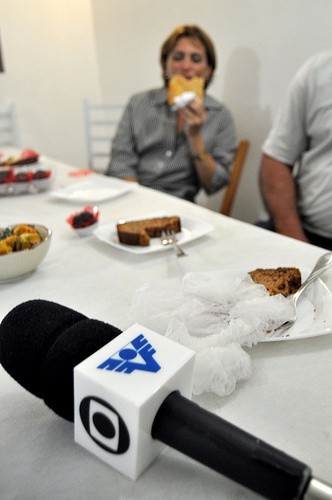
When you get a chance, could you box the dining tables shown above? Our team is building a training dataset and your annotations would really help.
[0,148,332,500]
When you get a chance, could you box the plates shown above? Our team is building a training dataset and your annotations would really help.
[256,268,332,342]
[48,176,138,206]
[93,211,213,253]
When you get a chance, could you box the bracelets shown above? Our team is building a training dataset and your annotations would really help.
[192,149,208,160]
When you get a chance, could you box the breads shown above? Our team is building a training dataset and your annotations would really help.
[167,73,204,105]
[116,217,180,246]
[247,267,301,298]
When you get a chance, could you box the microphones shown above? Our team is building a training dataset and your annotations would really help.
[0,299,332,500]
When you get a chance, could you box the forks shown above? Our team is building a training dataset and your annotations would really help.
[265,252,332,334]
[161,229,188,257]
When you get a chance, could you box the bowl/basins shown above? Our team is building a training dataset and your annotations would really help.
[0,224,52,284]
[66,206,99,237]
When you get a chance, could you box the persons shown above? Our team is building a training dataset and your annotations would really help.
[255,49,332,252]
[104,23,238,203]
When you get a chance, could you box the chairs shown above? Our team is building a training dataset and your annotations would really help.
[192,140,251,218]
[83,101,133,173]
